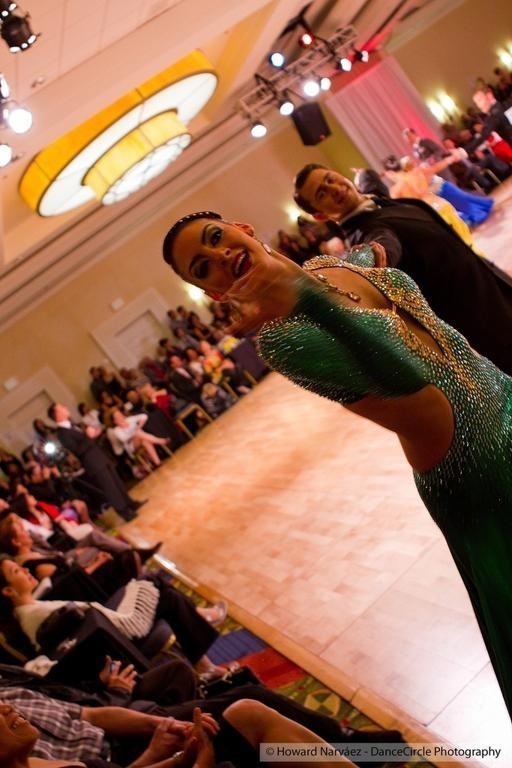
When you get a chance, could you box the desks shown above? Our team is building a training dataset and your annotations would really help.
[35,532,156,693]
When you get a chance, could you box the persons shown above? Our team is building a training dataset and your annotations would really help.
[162,211,510,723]
[0,400,406,767]
[88,60,510,400]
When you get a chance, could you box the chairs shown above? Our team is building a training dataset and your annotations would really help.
[139,618,186,664]
[468,166,501,197]
[133,365,259,474]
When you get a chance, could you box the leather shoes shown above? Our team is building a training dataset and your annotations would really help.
[141,541,163,566]
[124,500,148,521]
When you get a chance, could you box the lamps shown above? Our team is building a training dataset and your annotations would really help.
[2,0,367,220]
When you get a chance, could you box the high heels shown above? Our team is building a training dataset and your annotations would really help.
[197,602,229,632]
[199,661,243,685]
